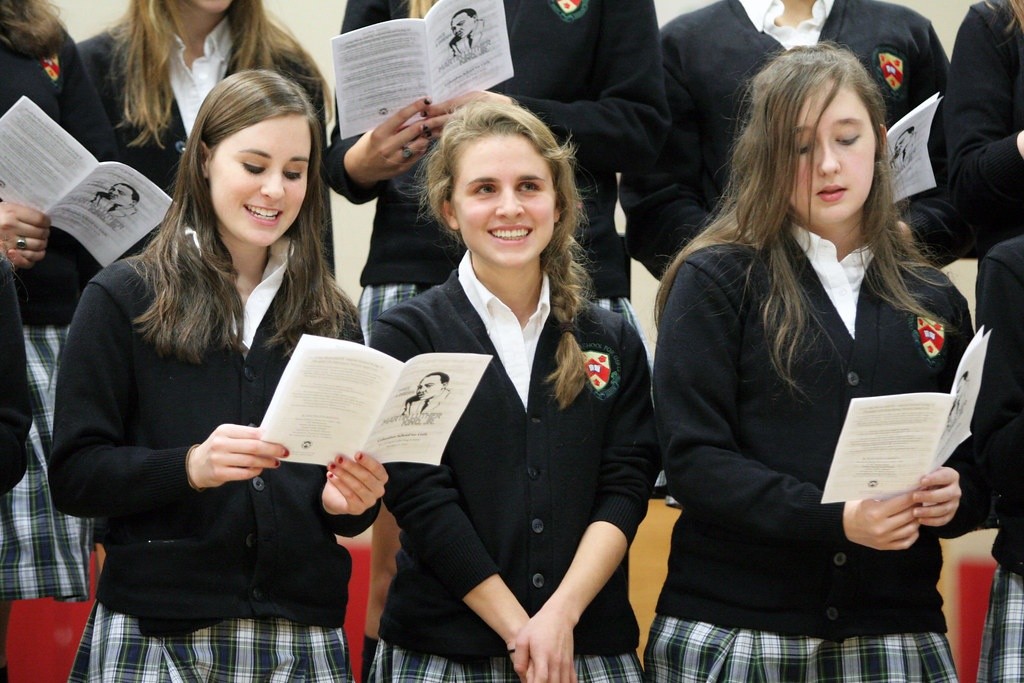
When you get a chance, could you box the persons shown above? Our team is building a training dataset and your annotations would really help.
[0,0,1024,683]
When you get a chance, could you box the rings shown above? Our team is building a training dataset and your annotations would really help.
[400,145,413,160]
[16,236,27,250]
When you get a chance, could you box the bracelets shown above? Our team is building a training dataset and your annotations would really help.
[506,648,515,653]
[186,443,207,492]
